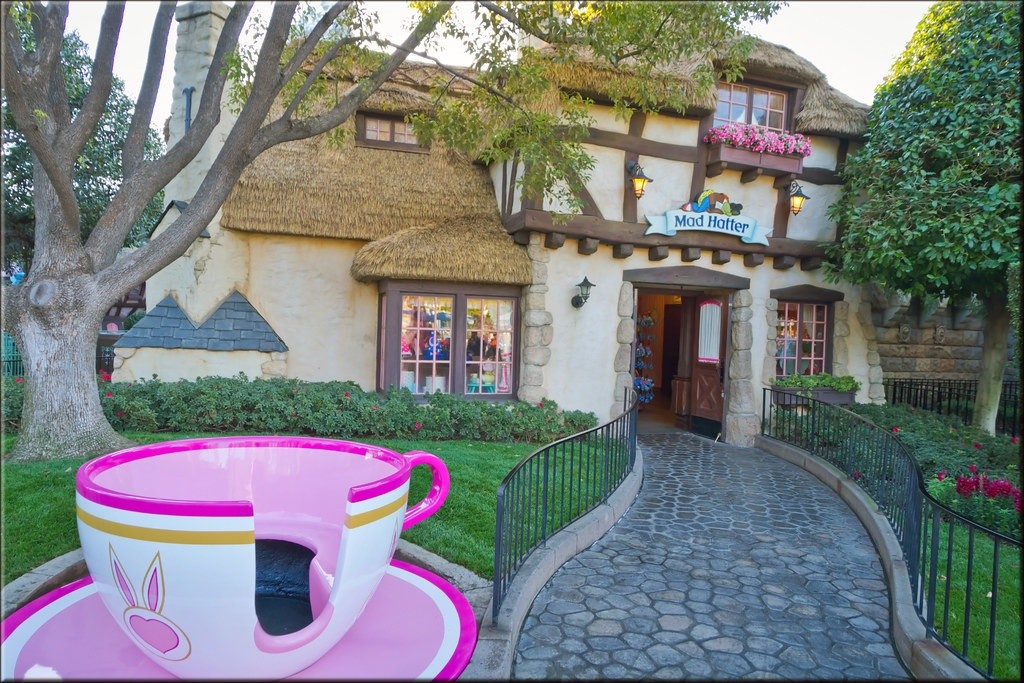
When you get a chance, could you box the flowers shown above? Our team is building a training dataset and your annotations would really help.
[702,122,811,158]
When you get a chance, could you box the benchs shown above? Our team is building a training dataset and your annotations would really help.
[253,515,342,622]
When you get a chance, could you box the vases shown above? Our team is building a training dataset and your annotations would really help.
[706,143,804,176]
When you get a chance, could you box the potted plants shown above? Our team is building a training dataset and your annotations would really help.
[769,372,863,405]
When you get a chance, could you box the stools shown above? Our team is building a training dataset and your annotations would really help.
[467,384,494,393]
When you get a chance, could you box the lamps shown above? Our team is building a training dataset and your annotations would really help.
[783,180,810,215]
[627,161,654,200]
[572,276,596,308]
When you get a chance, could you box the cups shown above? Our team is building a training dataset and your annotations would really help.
[470,374,478,384]
